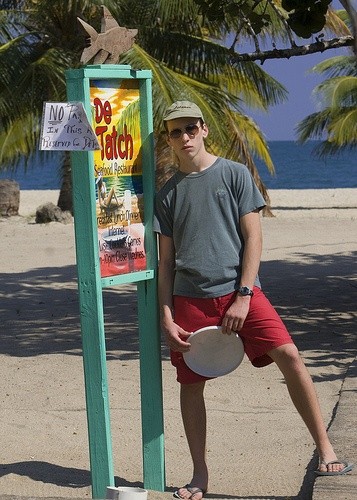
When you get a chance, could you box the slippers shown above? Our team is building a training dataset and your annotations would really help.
[313,460,355,476]
[174,484,207,500]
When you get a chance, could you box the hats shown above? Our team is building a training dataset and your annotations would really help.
[163,100,205,124]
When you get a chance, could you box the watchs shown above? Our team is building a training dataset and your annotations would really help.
[237,286,254,297]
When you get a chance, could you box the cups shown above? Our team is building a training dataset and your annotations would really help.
[100,228,128,248]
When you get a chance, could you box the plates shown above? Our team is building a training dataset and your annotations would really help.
[183,326,244,378]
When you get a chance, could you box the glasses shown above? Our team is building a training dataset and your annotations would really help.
[167,124,200,139]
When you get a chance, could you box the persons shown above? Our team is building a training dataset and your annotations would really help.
[98,179,121,208]
[151,101,353,500]
